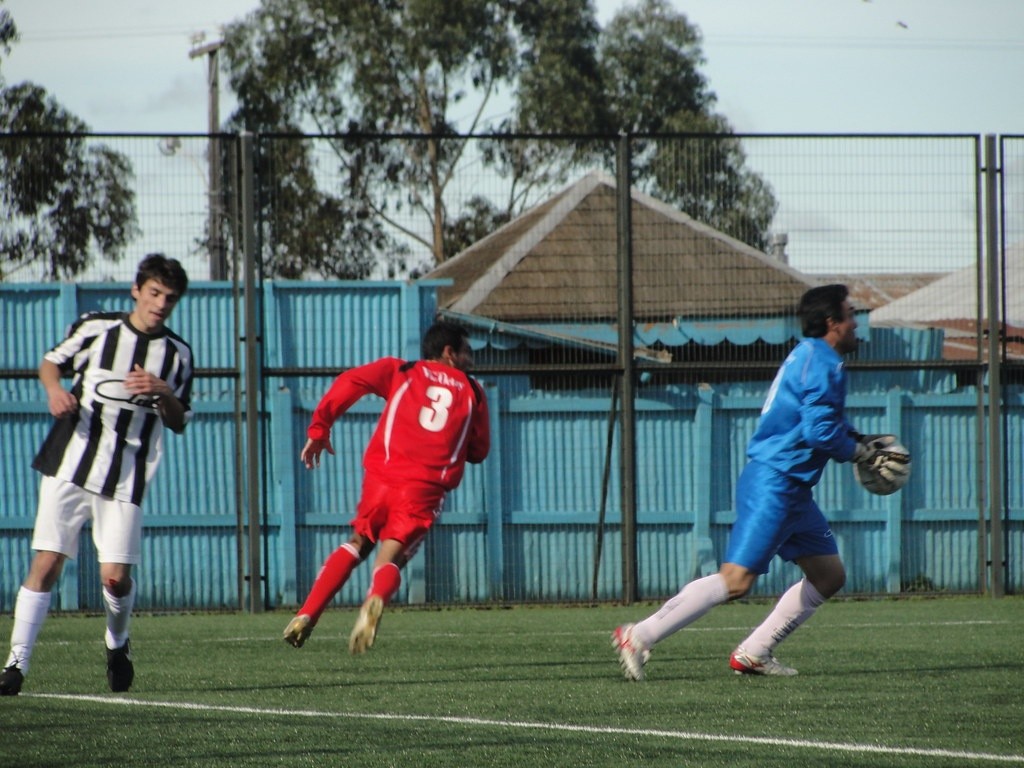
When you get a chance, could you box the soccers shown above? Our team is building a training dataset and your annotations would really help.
[853,433,913,494]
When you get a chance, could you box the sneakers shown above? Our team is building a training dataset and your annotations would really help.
[105,637,134,692]
[610,622,652,682]
[349,596,384,655]
[0,660,23,696]
[729,645,799,676]
[283,614,313,648]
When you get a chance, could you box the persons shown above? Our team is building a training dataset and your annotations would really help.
[607,283,909,680]
[1,253,194,696]
[283,320,491,650]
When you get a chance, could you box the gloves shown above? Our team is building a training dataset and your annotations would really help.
[848,434,912,482]
